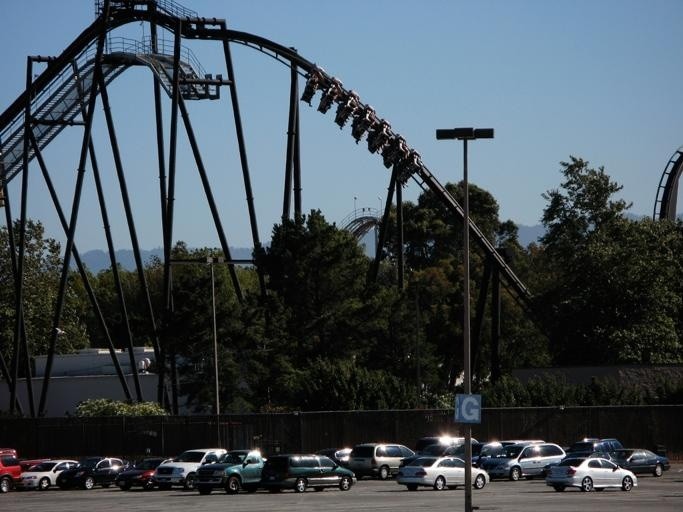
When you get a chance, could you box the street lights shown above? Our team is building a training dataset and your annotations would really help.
[379,196,383,217]
[434,125,496,511]
[166,251,258,446]
[352,195,359,221]
